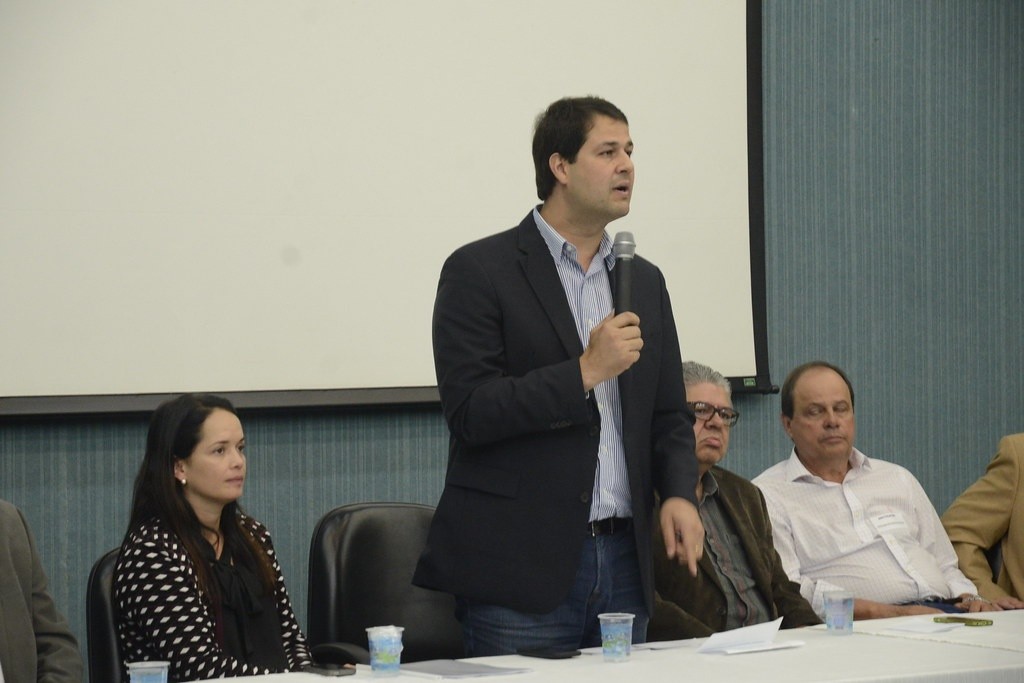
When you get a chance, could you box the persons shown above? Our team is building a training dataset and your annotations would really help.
[941,432,1024,611]
[412,96,705,660]
[750,360,995,621]
[645,360,825,643]
[111,395,313,683]
[0,500,82,683]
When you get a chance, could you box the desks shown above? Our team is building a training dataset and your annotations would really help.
[185,608,1024,682]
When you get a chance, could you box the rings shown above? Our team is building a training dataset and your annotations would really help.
[695,543,701,551]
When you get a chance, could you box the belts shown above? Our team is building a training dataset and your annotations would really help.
[586,518,633,538]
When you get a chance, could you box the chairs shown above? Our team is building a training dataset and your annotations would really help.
[85,548,127,683]
[305,502,465,665]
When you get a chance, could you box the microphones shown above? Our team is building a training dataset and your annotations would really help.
[612,230,636,327]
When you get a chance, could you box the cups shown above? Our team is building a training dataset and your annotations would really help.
[822,590,855,634]
[126,661,170,683]
[598,613,635,662]
[366,626,404,677]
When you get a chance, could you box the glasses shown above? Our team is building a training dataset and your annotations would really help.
[687,401,739,427]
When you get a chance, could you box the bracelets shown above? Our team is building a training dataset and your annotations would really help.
[962,595,992,606]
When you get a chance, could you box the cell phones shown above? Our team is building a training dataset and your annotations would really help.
[304,663,356,678]
[933,616,993,626]
